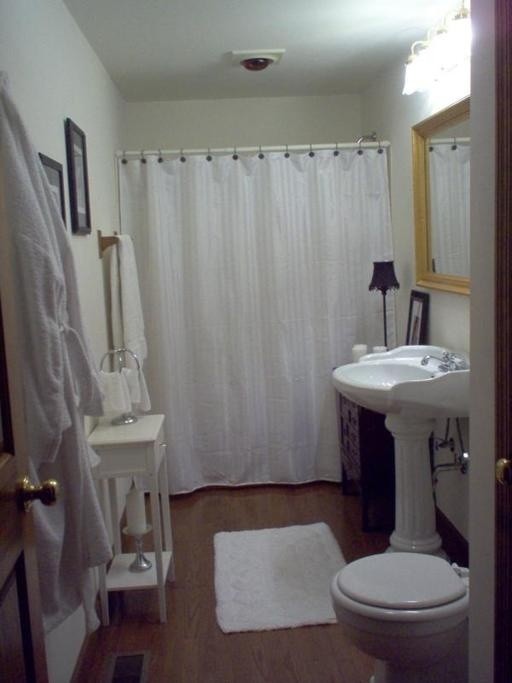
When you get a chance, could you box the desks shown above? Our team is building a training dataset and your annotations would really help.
[89,414,176,626]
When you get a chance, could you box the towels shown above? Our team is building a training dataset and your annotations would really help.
[100,371,129,413]
[120,364,151,413]
[111,233,149,369]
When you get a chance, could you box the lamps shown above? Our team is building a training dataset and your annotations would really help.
[403,0,471,96]
[369,261,400,346]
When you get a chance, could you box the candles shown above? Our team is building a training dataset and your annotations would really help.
[126,473,145,530]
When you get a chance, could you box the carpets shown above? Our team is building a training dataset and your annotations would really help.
[214,521,347,631]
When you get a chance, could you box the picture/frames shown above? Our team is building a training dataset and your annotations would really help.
[38,151,66,236]
[407,290,426,343]
[65,116,93,236]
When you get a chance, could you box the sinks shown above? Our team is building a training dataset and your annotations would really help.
[333,344,470,407]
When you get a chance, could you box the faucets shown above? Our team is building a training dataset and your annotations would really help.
[421,347,464,372]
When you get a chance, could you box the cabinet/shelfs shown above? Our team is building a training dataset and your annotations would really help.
[333,362,438,533]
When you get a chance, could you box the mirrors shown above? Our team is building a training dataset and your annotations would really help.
[410,95,473,296]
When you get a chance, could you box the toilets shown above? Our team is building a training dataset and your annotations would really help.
[330,552,470,682]
